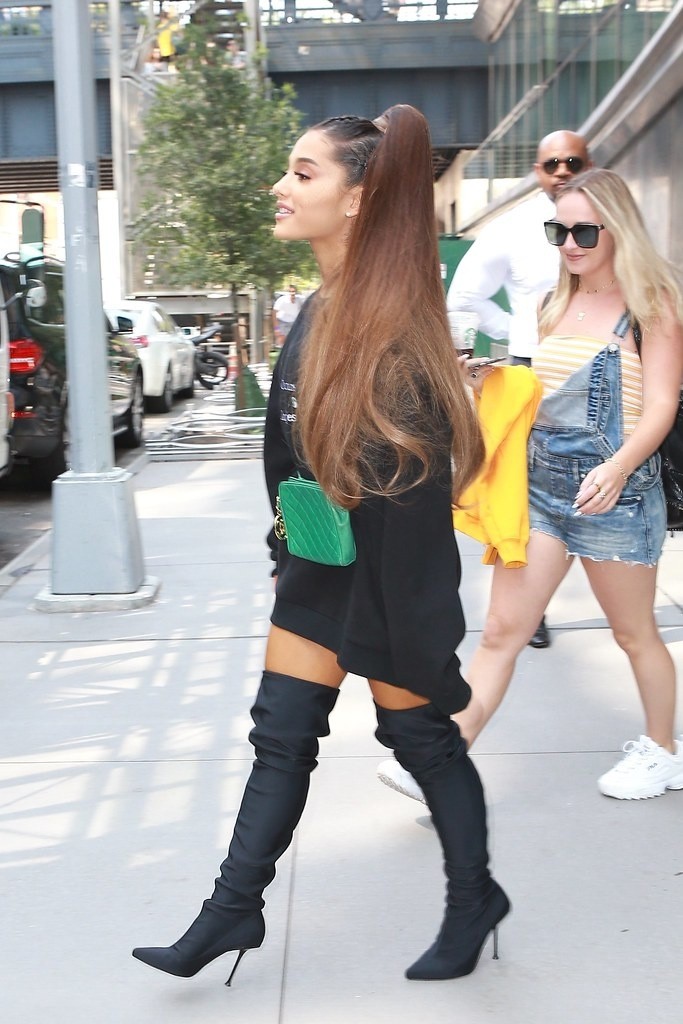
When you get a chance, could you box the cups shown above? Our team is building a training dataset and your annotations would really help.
[448,312,479,360]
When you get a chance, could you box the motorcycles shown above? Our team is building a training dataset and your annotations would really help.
[190,328,229,388]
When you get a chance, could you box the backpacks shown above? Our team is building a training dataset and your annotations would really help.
[540,289,683,531]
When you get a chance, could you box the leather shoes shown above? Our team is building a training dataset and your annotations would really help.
[528,621,549,648]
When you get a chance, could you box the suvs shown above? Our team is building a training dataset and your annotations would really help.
[0,252,144,489]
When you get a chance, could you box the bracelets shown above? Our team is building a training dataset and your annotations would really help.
[604,458,628,485]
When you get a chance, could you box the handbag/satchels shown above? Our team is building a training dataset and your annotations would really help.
[273,476,357,567]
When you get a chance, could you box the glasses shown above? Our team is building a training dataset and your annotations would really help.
[543,220,605,248]
[540,156,583,174]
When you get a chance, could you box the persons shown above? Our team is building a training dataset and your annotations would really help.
[445,130,596,648]
[379,0,400,21]
[145,11,245,73]
[377,168,683,805]
[132,103,512,985]
[272,285,307,345]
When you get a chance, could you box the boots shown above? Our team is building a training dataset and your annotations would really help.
[373,698,510,980]
[131,670,342,986]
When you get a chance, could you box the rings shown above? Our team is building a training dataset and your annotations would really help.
[597,491,607,500]
[592,482,601,492]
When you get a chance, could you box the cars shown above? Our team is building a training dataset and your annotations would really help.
[103,300,201,413]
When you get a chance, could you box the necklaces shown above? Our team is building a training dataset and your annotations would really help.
[576,275,617,321]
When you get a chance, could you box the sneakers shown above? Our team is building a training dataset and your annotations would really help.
[376,762,428,807]
[597,736,682,800]
